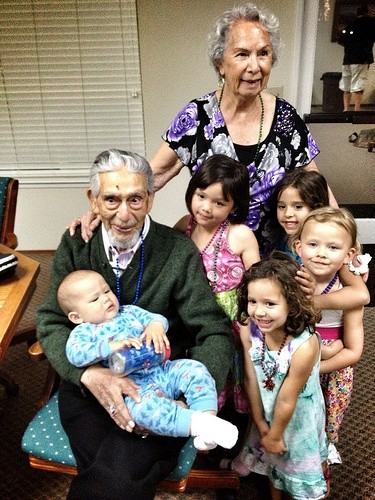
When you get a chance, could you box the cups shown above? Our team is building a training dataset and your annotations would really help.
[109,339,170,378]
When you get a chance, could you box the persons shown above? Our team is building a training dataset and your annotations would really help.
[57,270,239,451]
[338,3,375,112]
[65,2,369,284]
[172,153,370,500]
[36,149,233,500]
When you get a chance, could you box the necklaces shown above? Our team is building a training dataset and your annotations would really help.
[218,82,264,162]
[190,220,228,290]
[321,272,339,294]
[116,234,145,305]
[262,332,289,390]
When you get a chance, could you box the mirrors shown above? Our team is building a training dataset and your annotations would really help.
[286,0,375,125]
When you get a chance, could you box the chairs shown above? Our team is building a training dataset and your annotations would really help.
[21,340,241,500]
[0,177,19,250]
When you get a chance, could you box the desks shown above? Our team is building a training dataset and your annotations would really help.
[0,243,40,362]
